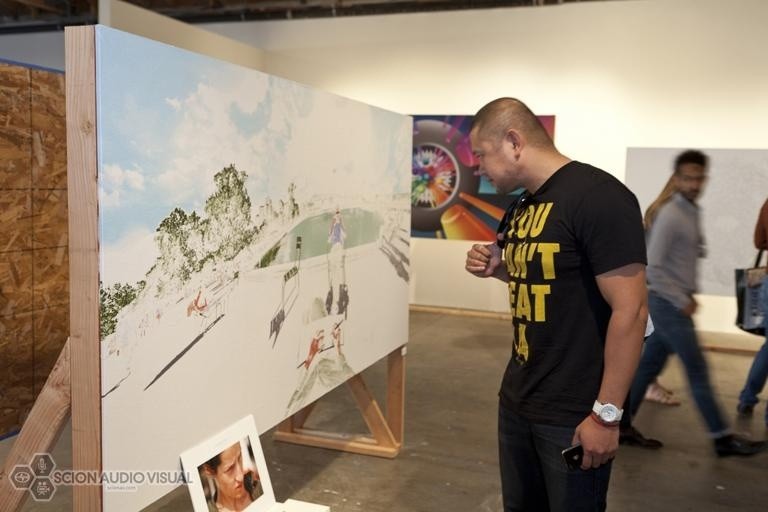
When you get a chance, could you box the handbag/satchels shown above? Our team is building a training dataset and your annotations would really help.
[735,266,768,336]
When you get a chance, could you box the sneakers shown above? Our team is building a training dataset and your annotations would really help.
[642,383,682,405]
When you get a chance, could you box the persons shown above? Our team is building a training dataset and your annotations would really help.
[199,441,263,512]
[463,96,649,512]
[641,169,683,407]
[615,150,768,459]
[736,193,768,418]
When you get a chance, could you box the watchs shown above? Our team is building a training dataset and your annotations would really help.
[593,400,624,424]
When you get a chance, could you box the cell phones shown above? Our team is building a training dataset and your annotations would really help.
[561,443,584,473]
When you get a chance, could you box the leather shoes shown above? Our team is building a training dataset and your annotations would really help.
[716,437,767,458]
[618,431,662,450]
